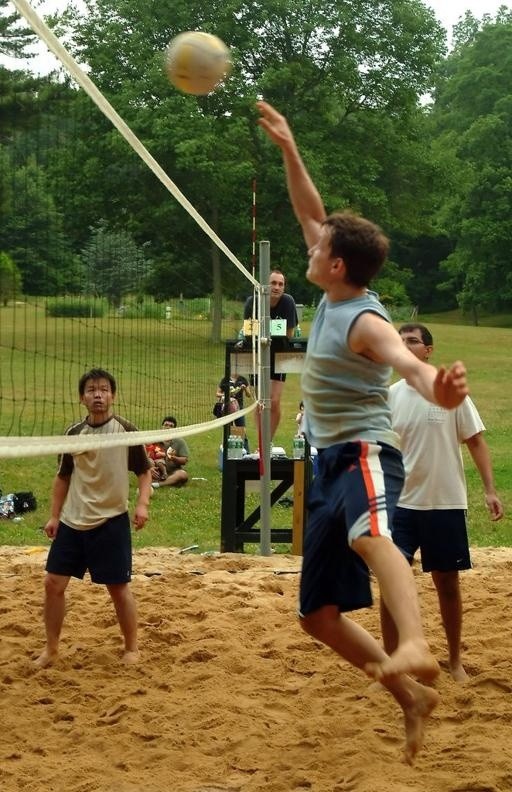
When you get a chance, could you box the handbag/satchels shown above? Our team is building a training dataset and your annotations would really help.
[1,491,37,519]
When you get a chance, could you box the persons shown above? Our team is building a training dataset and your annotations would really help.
[136,414,191,498]
[294,401,306,432]
[378,321,506,687]
[214,369,254,456]
[250,94,474,766]
[243,269,301,457]
[146,441,168,481]
[25,366,158,673]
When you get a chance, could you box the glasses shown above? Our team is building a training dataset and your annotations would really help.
[401,337,427,347]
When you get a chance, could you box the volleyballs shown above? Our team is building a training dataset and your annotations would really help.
[168,32,233,93]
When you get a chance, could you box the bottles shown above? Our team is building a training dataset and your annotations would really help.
[292,435,306,460]
[228,435,243,459]
